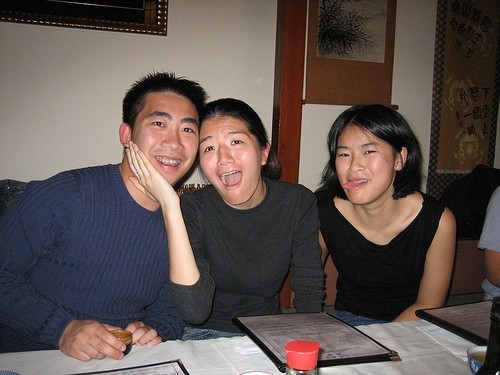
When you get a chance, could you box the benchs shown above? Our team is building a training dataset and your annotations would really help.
[324,240,483,300]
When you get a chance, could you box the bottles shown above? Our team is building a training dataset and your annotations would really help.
[283,341,320,375]
[476,296,500,375]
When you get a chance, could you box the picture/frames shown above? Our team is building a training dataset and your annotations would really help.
[0,0,168,36]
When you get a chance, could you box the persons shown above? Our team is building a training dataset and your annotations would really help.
[0,72,207,362]
[315,104,456,327]
[126,98,326,340]
[478,185,500,301]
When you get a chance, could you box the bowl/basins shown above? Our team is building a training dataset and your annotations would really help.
[467,345,487,375]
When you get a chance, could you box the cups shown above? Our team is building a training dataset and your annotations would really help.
[109,329,132,357]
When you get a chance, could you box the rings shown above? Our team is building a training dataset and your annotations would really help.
[136,168,140,172]
